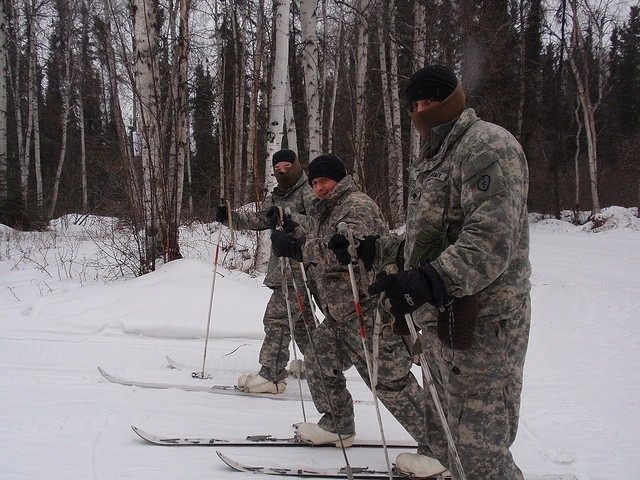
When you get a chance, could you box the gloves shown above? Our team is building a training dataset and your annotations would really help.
[328,233,380,271]
[368,261,448,315]
[265,205,300,233]
[216,207,227,222]
[270,230,306,262]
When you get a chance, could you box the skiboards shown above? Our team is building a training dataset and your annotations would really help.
[131,425,452,480]
[98,356,381,405]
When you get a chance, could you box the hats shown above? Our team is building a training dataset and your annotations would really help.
[308,154,346,188]
[273,149,295,168]
[406,64,457,104]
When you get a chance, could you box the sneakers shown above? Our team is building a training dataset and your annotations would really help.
[298,422,356,447]
[290,359,307,380]
[238,373,287,394]
[396,452,451,477]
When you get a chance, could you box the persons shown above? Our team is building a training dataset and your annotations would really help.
[216,150,321,395]
[269,152,448,477]
[327,64,532,480]
